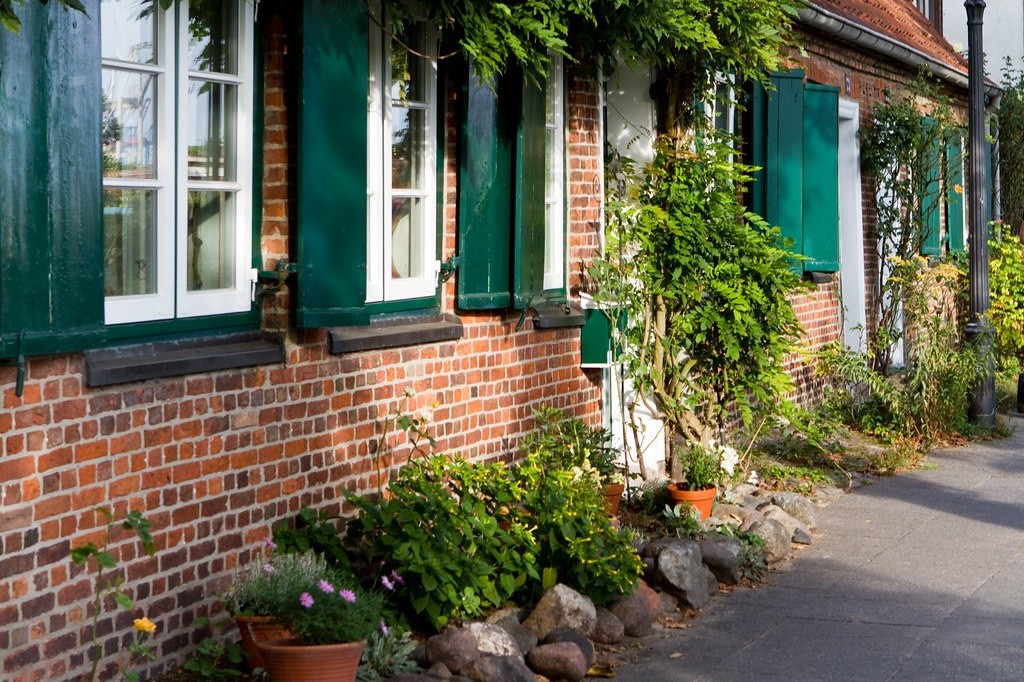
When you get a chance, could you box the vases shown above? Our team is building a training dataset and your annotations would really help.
[233,616,298,672]
[259,638,368,682]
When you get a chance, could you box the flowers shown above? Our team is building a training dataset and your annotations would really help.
[225,537,403,644]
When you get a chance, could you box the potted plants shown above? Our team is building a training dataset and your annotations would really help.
[590,427,624,517]
[668,441,725,524]
